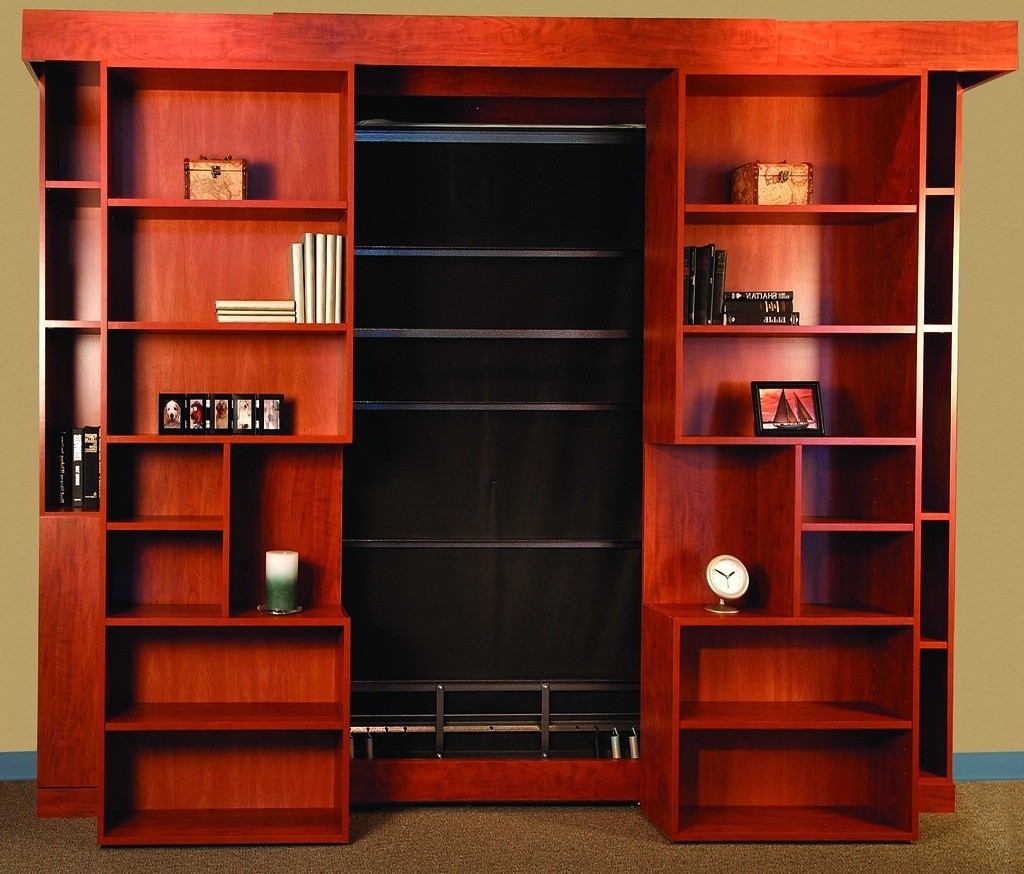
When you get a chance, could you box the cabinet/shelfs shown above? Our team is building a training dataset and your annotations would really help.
[21,10,1017,845]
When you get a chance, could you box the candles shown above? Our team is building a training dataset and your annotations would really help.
[264,550,299,612]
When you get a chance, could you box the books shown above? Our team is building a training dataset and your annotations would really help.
[57,422,101,512]
[216,230,346,323]
[682,242,801,326]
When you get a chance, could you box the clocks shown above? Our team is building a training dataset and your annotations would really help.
[704,555,750,614]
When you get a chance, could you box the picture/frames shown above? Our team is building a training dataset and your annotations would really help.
[751,381,825,436]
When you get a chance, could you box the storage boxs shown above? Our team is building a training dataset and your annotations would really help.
[184,156,248,200]
[731,161,813,205]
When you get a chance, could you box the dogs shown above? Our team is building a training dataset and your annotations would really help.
[164,400,181,428]
[264,400,280,429]
[216,400,228,429]
[237,400,252,429]
[190,399,203,429]
